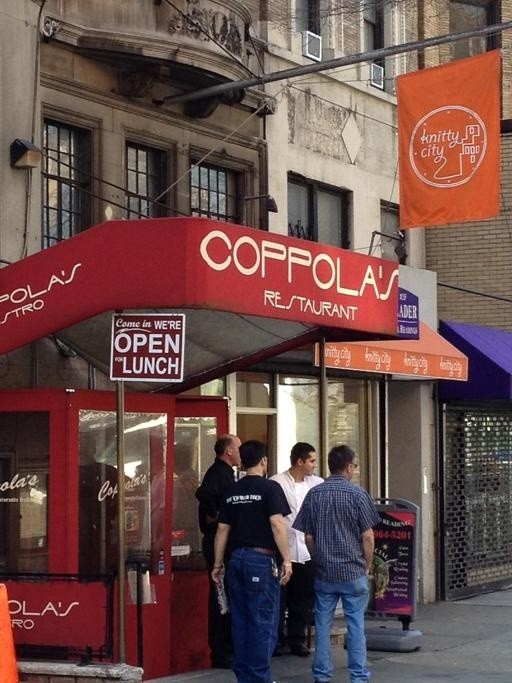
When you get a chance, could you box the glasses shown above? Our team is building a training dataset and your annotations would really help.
[348,462,358,469]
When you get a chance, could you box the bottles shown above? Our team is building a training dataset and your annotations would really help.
[158,549,165,576]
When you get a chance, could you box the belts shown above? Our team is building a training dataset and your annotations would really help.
[244,547,273,554]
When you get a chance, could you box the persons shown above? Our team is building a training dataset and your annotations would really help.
[268,442,326,656]
[171,442,199,552]
[140,441,186,552]
[45,430,143,572]
[292,444,382,683]
[197,434,243,669]
[211,440,293,682]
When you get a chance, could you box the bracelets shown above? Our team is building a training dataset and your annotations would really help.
[212,563,226,570]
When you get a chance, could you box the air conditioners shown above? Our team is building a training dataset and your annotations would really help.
[369,63,384,90]
[301,30,322,63]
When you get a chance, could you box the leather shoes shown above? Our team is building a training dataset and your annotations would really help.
[289,641,311,657]
[272,642,284,656]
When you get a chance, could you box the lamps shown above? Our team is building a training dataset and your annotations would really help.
[246,195,278,213]
